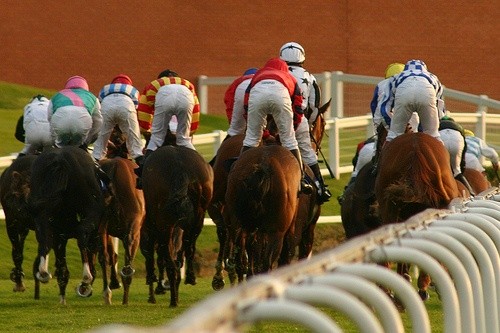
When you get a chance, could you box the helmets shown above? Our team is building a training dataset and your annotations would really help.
[384,62,405,78]
[159,69,179,77]
[112,73,133,85]
[64,75,89,91]
[464,129,475,136]
[31,95,48,103]
[244,68,257,75]
[279,41,306,63]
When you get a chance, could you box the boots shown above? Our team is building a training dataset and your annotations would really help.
[290,149,314,192]
[306,162,332,202]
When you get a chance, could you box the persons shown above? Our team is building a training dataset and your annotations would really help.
[237,58,314,195]
[371,58,445,179]
[207,42,331,204]
[15,74,177,189]
[351,62,497,198]
[137,69,201,165]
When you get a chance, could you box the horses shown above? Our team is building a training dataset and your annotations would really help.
[207,133,318,286]
[380,132,454,308]
[341,155,489,310]
[131,143,217,309]
[98,151,142,290]
[0,144,125,295]
